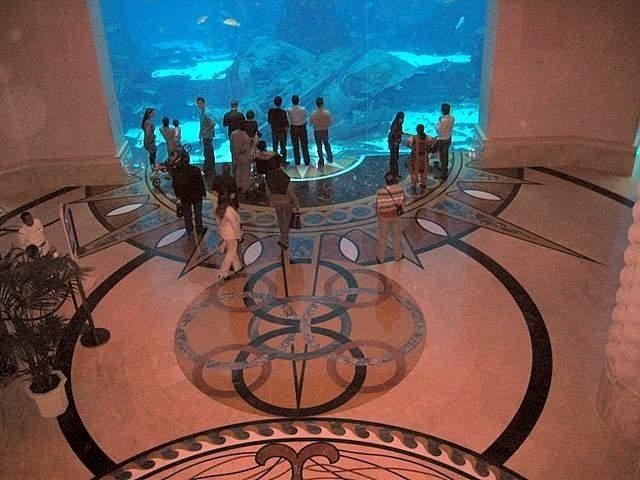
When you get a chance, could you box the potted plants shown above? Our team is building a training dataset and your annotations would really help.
[0,239,111,418]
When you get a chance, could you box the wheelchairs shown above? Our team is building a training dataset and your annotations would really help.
[250,152,284,191]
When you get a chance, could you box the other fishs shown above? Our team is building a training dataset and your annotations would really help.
[455,16,465,30]
[224,18,240,29]
[197,16,208,24]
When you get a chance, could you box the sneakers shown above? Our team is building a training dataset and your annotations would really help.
[219,267,244,280]
[276,241,288,249]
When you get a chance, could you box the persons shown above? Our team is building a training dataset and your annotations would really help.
[432,103,455,180]
[374,171,408,264]
[141,96,302,282]
[403,123,439,192]
[25,243,41,263]
[308,96,335,167]
[386,110,405,178]
[286,94,311,166]
[16,210,59,258]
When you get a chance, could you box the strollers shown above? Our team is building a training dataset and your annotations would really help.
[150,142,193,188]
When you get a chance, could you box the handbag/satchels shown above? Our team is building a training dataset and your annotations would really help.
[290,211,301,230]
[397,205,403,216]
[176,202,184,217]
